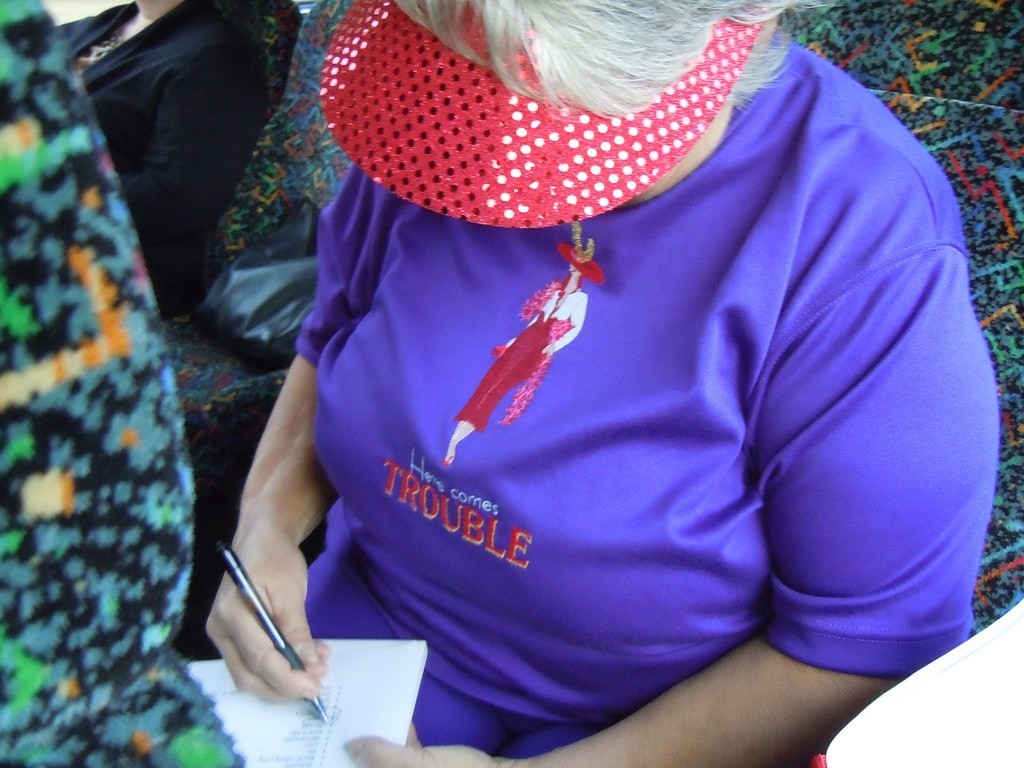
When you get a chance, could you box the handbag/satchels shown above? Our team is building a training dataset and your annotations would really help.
[192,201,327,371]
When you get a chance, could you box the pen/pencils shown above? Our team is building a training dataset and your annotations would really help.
[215,539,329,723]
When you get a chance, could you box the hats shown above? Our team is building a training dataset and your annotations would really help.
[318,0,768,229]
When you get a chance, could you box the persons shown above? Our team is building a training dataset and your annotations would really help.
[0,0,302,768]
[203,0,1000,768]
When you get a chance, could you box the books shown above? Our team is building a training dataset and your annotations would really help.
[187,640,429,768]
